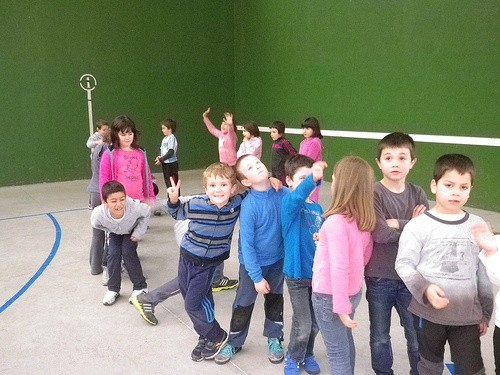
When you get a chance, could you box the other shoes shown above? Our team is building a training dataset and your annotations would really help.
[103,266,109,286]
[88,203,92,212]
[153,209,161,216]
[120,260,128,277]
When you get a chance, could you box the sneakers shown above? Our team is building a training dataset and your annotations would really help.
[201,327,228,359]
[267,337,284,364]
[212,276,239,291]
[214,343,242,364]
[284,351,301,375]
[131,293,158,326]
[302,354,320,375]
[102,290,120,305]
[190,335,207,362]
[129,288,150,305]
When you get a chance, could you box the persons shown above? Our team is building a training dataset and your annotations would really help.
[100,115,155,286]
[214,152,285,365]
[90,130,113,276]
[167,162,283,362]
[128,181,248,327]
[87,118,109,211]
[203,107,238,168]
[90,181,152,306]
[280,152,328,375]
[154,119,181,198]
[311,156,375,375]
[363,131,429,375]
[473,223,500,375]
[238,121,262,161]
[394,153,493,375]
[298,117,324,202]
[269,120,298,188]
